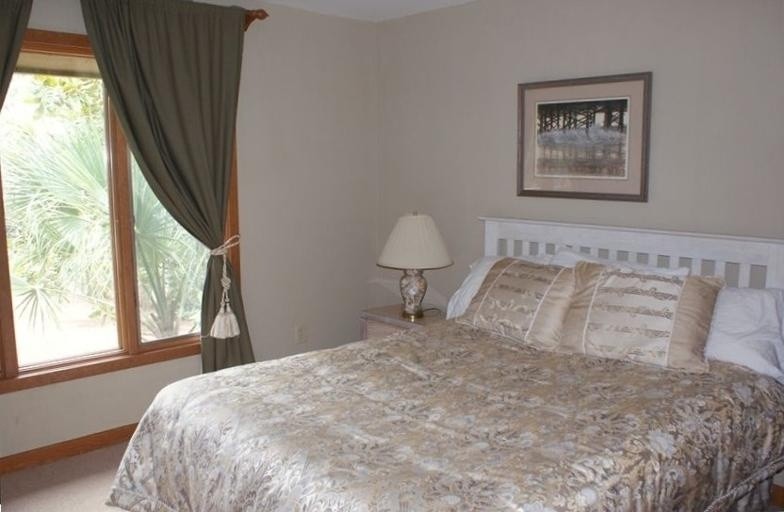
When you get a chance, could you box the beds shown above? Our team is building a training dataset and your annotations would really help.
[115,216,784,508]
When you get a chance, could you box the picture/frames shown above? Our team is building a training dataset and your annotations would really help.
[512,70,657,206]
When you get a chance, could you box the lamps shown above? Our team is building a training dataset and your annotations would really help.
[375,207,453,319]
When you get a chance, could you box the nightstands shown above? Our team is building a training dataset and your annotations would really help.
[358,301,443,338]
